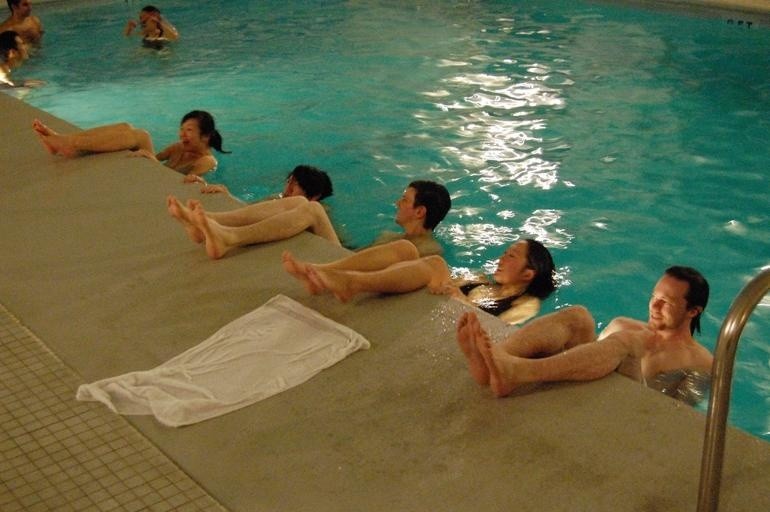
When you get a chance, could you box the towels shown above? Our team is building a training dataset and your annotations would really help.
[74,293,371,427]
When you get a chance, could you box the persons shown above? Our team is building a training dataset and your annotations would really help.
[32,110,232,180]
[0,1,43,42]
[125,6,178,51]
[1,30,47,101]
[281,239,558,326]
[457,265,717,408]
[167,179,452,261]
[182,165,334,203]
